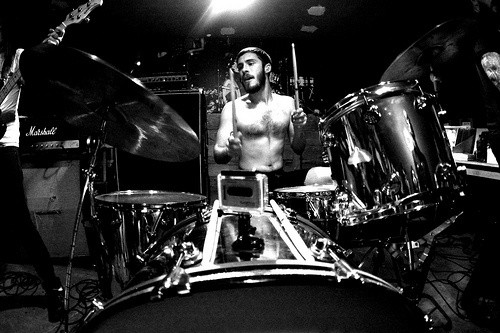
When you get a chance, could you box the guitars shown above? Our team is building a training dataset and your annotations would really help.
[0,0,105,107]
[64,200,438,333]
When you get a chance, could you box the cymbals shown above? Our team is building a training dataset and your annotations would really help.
[17,42,203,152]
[380,14,477,86]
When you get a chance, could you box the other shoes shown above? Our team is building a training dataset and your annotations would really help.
[45,286,65,322]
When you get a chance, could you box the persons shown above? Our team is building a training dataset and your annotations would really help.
[214,46,334,200]
[0,26,67,324]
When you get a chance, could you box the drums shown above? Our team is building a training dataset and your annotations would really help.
[274,184,338,239]
[314,76,471,246]
[95,190,207,289]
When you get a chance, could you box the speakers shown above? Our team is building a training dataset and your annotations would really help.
[20,152,94,263]
[118,90,206,196]
